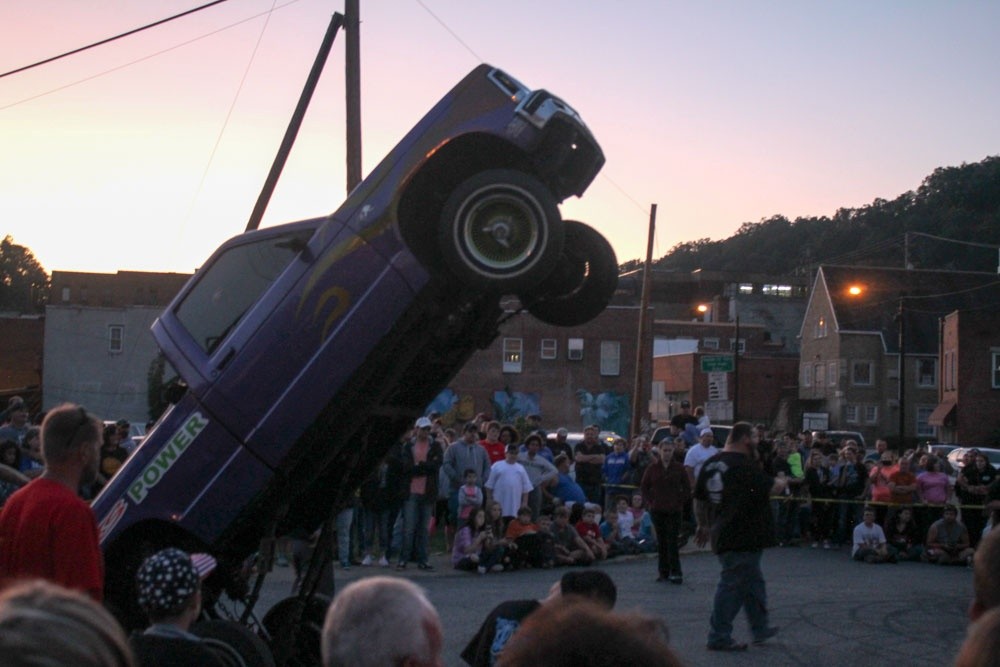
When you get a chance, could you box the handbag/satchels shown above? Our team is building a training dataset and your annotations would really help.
[650,495,671,524]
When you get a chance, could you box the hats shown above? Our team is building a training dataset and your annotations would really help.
[681,400,690,408]
[563,570,617,611]
[779,442,789,449]
[558,428,568,437]
[943,505,957,513]
[414,417,432,428]
[8,396,27,412]
[118,419,131,427]
[504,444,516,454]
[700,428,712,437]
[137,547,217,612]
[668,415,685,431]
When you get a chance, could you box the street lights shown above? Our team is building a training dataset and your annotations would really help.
[697,300,739,426]
[848,286,906,459]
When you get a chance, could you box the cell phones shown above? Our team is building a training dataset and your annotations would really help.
[883,461,892,466]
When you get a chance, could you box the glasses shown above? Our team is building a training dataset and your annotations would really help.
[63,408,89,448]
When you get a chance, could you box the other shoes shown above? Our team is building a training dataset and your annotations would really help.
[707,638,748,651]
[667,574,683,584]
[350,558,361,566]
[362,556,373,567]
[773,537,853,552]
[396,562,406,570]
[342,563,350,570]
[656,572,664,582]
[417,562,432,570]
[753,626,779,644]
[378,557,389,567]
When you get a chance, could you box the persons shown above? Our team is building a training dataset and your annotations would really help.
[693,421,781,651]
[136,548,218,639]
[0,404,104,596]
[460,571,616,667]
[339,410,654,574]
[641,436,690,584]
[955,524,999,666]
[319,577,446,667]
[81,417,155,500]
[671,400,697,431]
[674,405,1000,563]
[650,419,682,445]
[495,594,685,667]
[0,581,132,667]
[0,396,47,504]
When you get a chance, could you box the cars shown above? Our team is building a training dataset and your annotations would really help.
[544,431,620,463]
[810,429,867,459]
[102,419,147,447]
[648,422,737,453]
[925,443,1000,492]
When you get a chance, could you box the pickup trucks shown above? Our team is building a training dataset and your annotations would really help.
[88,62,621,667]
